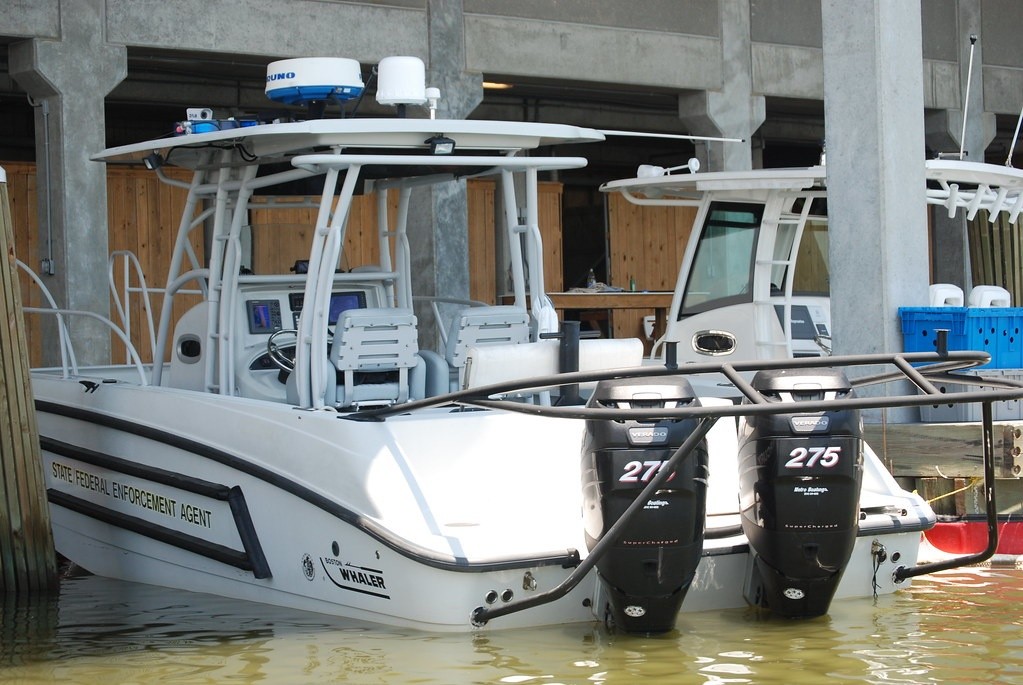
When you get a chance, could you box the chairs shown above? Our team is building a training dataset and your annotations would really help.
[284,306,426,412]
[416,304,531,407]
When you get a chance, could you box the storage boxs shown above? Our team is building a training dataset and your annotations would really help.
[898,306,1023,369]
[916,369,1023,421]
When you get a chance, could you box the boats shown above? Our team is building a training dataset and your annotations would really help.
[595,35,1022,409]
[26,52,1020,643]
